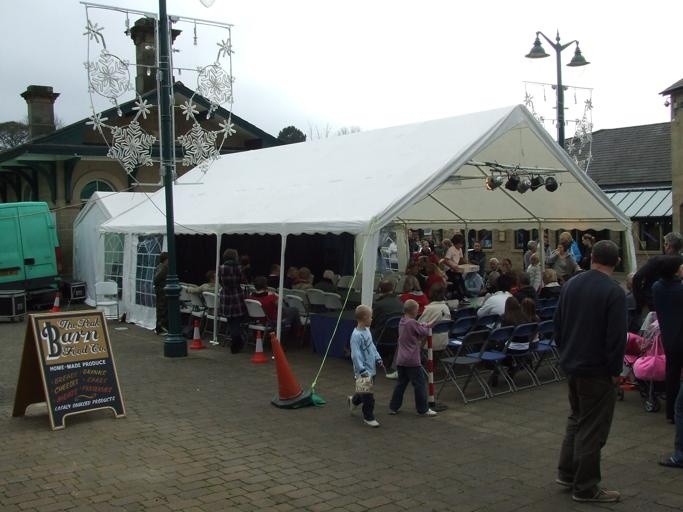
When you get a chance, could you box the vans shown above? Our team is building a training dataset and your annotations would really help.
[0,202,60,309]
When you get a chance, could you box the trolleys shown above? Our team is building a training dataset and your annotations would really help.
[0,289,26,323]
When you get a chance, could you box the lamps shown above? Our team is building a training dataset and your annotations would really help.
[477,163,565,193]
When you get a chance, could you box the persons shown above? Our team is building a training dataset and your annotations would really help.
[153,252,168,335]
[386,300,438,417]
[180,248,334,353]
[624,232,682,468]
[554,239,628,503]
[373,229,594,379]
[346,305,383,427]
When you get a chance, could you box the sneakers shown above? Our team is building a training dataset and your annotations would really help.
[556,477,620,503]
[346,370,437,427]
[660,455,683,468]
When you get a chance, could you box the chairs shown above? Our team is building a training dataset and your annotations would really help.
[178,268,563,351]
[540,335,560,362]
[92,280,120,325]
[447,316,480,380]
[418,321,451,380]
[530,318,559,389]
[434,330,489,406]
[466,324,514,403]
[494,322,538,391]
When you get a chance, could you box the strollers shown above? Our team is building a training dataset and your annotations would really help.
[616,310,666,414]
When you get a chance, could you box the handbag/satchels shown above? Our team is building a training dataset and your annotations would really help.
[632,335,666,382]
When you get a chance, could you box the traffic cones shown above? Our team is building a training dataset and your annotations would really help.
[188,320,206,349]
[50,293,61,311]
[251,330,267,365]
[267,330,313,410]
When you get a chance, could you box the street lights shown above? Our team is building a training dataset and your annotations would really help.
[523,26,588,149]
[484,165,559,194]
